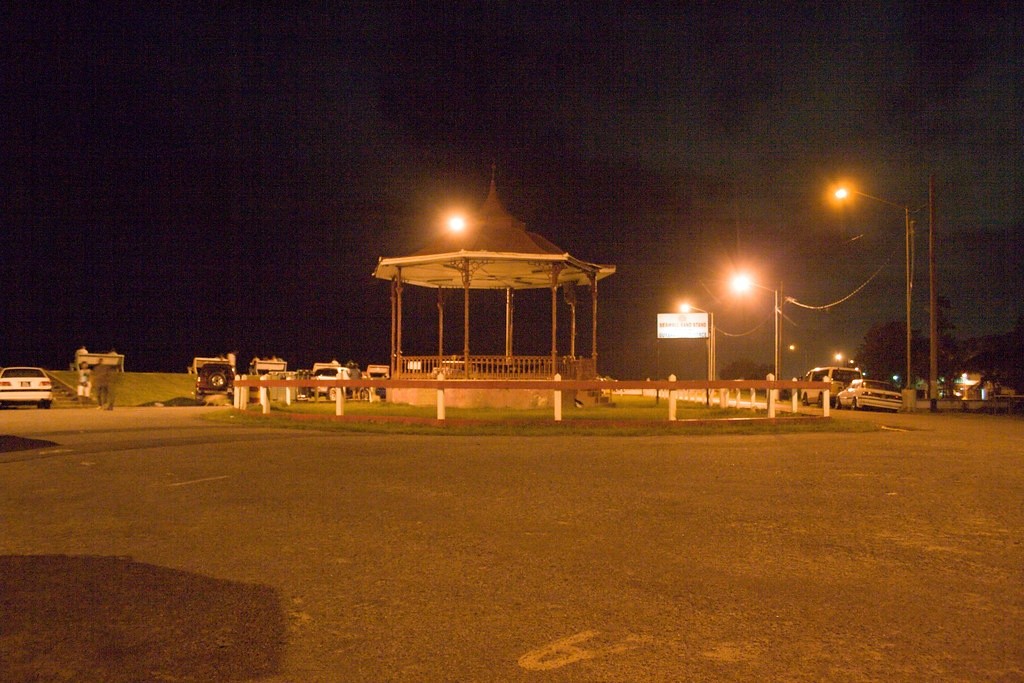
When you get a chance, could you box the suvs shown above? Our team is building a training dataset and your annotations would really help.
[195,363,235,398]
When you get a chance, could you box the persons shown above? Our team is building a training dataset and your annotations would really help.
[346,363,362,400]
[75,361,92,408]
[90,358,110,410]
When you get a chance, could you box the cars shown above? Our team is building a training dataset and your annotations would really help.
[433,361,478,375]
[0,367,54,409]
[307,367,372,401]
[836,378,903,413]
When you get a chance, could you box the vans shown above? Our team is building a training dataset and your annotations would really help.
[800,366,862,408]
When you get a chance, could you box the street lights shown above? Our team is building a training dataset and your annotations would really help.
[679,301,713,382]
[834,187,917,413]
[733,276,780,381]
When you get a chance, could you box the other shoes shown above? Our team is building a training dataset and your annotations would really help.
[96,406,103,410]
[105,405,114,410]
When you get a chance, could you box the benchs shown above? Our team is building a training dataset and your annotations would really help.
[929,399,989,415]
